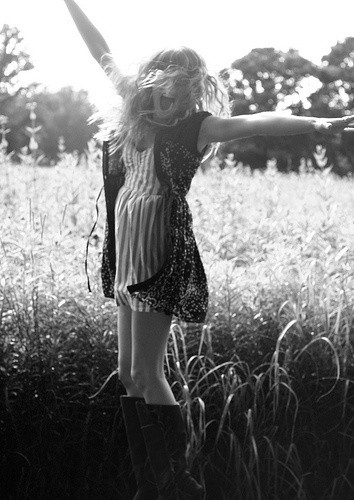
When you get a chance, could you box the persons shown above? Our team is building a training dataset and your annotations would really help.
[65,1,354,500]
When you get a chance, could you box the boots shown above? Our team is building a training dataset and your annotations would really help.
[135,400,204,500]
[119,394,158,500]
